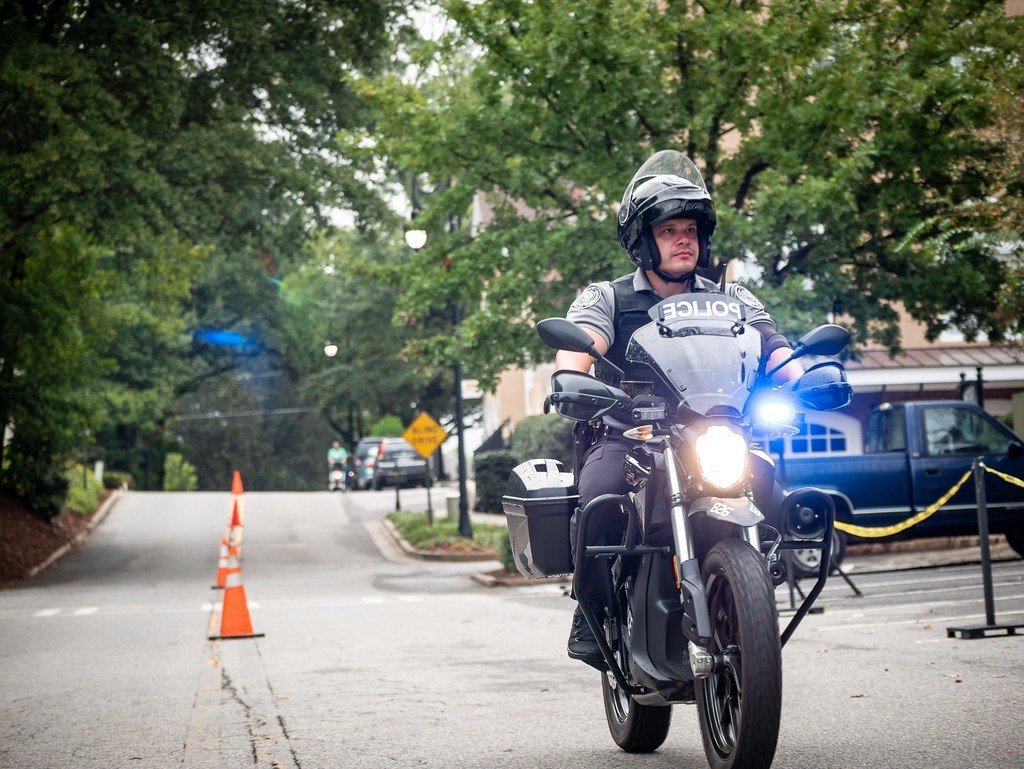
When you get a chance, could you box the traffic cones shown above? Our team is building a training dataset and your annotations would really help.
[211,535,232,590]
[232,470,243,494]
[207,542,267,640]
[231,500,243,528]
[226,530,241,561]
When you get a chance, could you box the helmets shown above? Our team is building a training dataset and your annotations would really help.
[618,150,716,283]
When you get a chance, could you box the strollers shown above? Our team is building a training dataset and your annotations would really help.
[329,462,347,493]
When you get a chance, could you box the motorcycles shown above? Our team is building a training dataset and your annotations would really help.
[501,313,852,769]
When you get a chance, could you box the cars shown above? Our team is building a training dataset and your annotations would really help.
[346,434,384,490]
[373,437,433,490]
[358,446,379,489]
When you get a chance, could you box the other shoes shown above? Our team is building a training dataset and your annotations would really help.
[568,604,604,659]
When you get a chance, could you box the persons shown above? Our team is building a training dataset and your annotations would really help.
[327,441,346,471]
[553,150,804,661]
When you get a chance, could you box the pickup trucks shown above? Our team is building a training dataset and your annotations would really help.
[770,397,1024,579]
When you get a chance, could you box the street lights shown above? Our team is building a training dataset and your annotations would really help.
[401,206,472,541]
